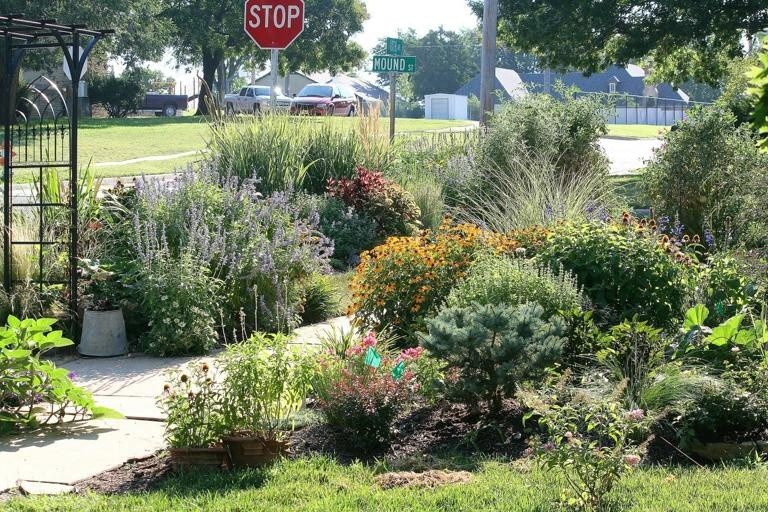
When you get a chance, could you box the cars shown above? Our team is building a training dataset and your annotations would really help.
[290,83,357,117]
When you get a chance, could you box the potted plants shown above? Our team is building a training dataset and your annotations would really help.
[76,280,130,357]
[215,329,314,469]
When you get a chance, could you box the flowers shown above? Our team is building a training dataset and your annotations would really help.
[154,363,229,447]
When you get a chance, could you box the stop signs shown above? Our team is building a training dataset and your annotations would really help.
[244,0,305,49]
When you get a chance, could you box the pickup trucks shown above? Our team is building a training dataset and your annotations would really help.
[137,95,188,116]
[224,85,292,115]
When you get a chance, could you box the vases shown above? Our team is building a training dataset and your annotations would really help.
[168,447,223,478]
